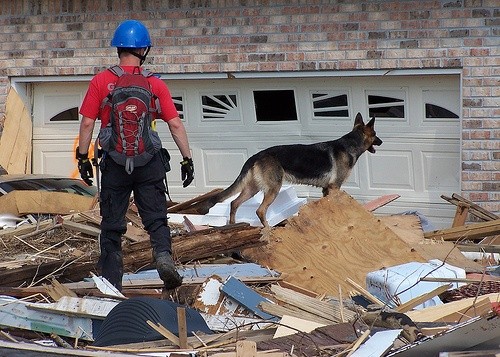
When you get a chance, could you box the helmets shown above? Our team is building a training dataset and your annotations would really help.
[110,19,152,47]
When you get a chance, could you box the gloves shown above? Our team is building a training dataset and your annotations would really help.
[78,157,93,186]
[180,157,195,188]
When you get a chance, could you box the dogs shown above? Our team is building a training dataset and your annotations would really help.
[208,111,383,228]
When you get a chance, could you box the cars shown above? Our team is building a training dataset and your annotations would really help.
[0,173,102,225]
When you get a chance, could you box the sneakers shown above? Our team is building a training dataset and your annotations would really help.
[153,251,182,290]
[100,267,122,294]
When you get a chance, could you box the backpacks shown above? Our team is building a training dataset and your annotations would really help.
[98,65,162,174]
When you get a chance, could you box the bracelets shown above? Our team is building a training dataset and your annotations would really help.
[76,146,88,159]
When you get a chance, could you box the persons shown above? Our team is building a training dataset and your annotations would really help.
[76,20,195,291]
